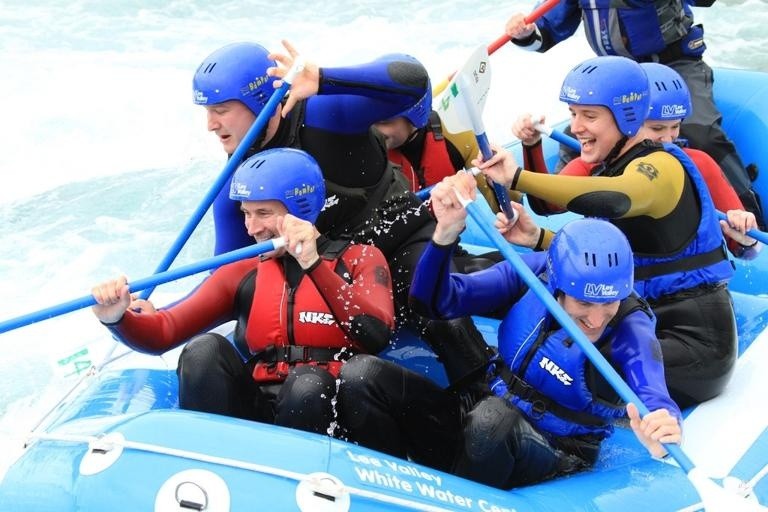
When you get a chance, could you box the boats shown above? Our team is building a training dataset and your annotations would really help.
[1,62,768,512]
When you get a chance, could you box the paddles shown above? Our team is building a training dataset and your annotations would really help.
[454,187,768,512]
[430,45,516,219]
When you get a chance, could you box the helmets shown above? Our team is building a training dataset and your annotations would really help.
[373,55,433,128]
[640,62,693,121]
[559,54,649,136]
[193,41,280,117]
[545,218,634,302]
[229,147,326,225]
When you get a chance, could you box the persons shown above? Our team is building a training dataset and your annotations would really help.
[471,55,738,408]
[371,54,524,213]
[337,169,683,491]
[504,0,768,232]
[126,40,496,386]
[91,148,395,438]
[512,63,763,260]
